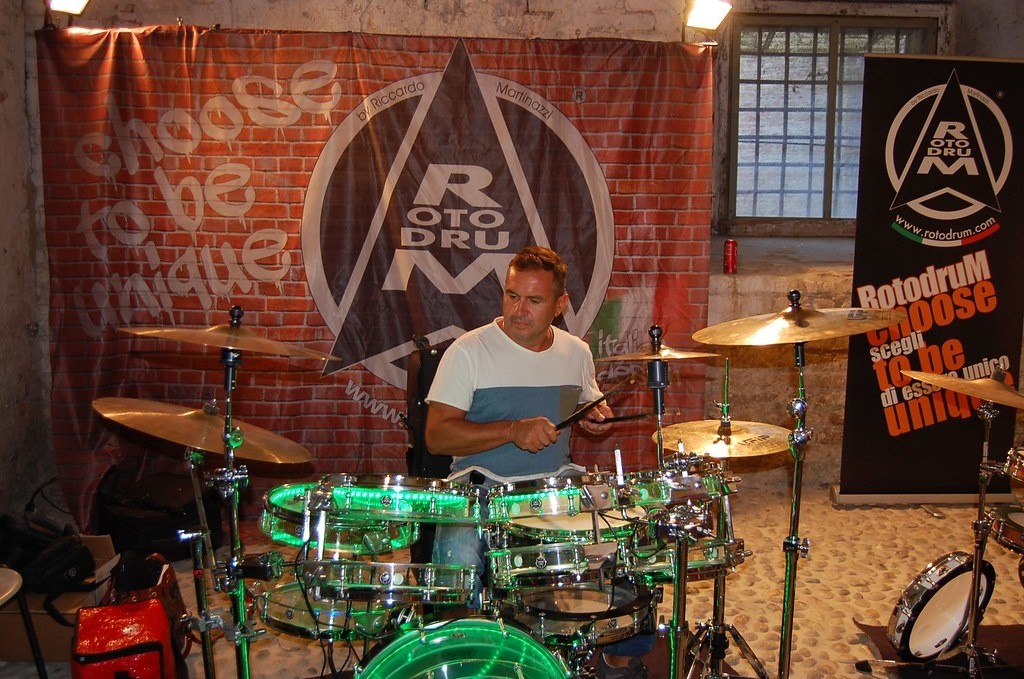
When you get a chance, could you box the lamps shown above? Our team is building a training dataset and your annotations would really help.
[43,0,89,29]
[681,0,733,47]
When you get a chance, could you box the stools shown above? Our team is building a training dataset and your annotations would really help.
[0,567,49,679]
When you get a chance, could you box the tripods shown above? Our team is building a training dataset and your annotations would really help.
[855,400,1024,679]
[683,459,771,679]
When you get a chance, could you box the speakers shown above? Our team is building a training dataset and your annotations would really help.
[406,347,453,479]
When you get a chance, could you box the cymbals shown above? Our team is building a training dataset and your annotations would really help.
[691,306,909,346]
[593,344,723,363]
[650,418,793,459]
[900,370,1024,411]
[115,319,344,362]
[90,398,314,465]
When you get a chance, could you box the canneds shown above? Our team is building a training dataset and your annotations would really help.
[723,239,738,274]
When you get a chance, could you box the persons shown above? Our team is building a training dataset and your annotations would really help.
[425,246,654,679]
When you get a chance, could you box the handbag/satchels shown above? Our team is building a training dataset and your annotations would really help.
[95,464,226,561]
[14,533,192,679]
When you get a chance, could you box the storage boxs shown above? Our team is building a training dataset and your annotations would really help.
[0,534,121,662]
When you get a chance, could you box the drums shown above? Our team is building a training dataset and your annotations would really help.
[246,463,737,679]
[884,445,1024,664]
